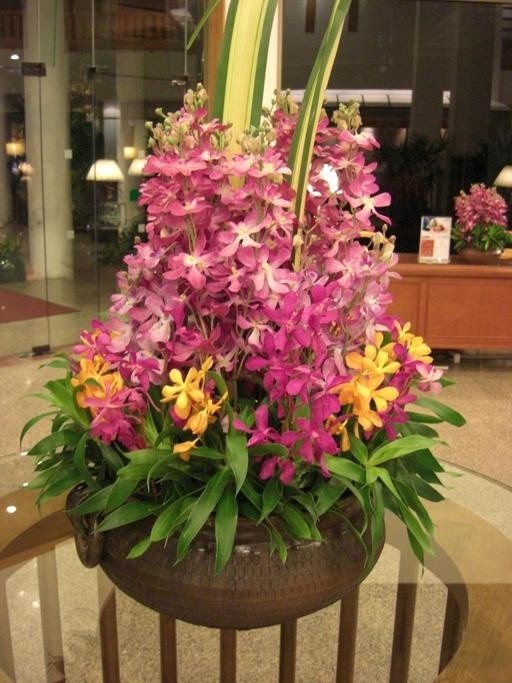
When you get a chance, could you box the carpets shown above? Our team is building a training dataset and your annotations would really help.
[1,285,80,325]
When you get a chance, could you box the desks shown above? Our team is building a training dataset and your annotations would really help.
[381,250,511,353]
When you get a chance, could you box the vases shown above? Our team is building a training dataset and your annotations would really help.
[458,244,504,264]
[61,483,391,635]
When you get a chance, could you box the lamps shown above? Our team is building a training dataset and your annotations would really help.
[86,157,126,199]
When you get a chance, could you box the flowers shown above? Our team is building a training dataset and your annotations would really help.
[14,79,474,590]
[450,180,511,253]
